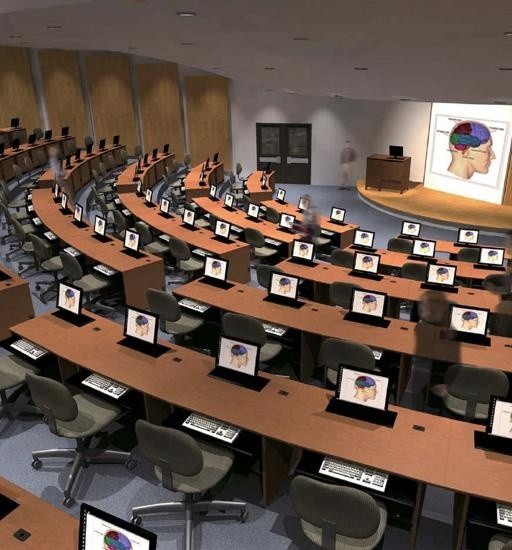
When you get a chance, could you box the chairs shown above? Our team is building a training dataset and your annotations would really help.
[128,418,248,549]
[24,372,137,509]
[2,127,512,430]
[289,477,389,549]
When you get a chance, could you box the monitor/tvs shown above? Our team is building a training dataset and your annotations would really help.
[179,208,200,232]
[420,262,458,293]
[10,119,20,130]
[473,394,512,456]
[135,180,145,197]
[198,254,235,291]
[325,362,399,428]
[71,202,90,228]
[78,502,157,550]
[440,303,491,346]
[207,335,271,392]
[116,305,172,357]
[53,183,62,203]
[287,239,318,267]
[157,197,175,219]
[343,288,390,328]
[145,188,157,208]
[407,238,438,262]
[210,220,236,244]
[350,228,377,253]
[2,126,219,185]
[389,146,404,160]
[90,215,114,243]
[330,206,346,225]
[297,197,312,216]
[59,191,74,215]
[276,212,296,234]
[120,228,149,259]
[397,220,422,240]
[246,202,263,223]
[264,163,273,174]
[473,246,506,271]
[454,228,480,248]
[274,187,288,205]
[262,271,307,310]
[348,251,384,281]
[50,280,95,327]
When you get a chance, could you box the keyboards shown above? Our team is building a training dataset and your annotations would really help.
[81,372,129,400]
[27,205,34,212]
[204,214,211,218]
[372,350,383,358]
[177,298,210,312]
[190,202,199,208]
[320,229,334,236]
[28,193,33,200]
[230,224,244,233]
[262,323,288,336]
[181,187,186,191]
[264,237,283,247]
[43,231,57,240]
[318,456,390,493]
[181,413,242,444]
[10,338,48,358]
[496,502,512,528]
[32,216,42,226]
[63,245,81,257]
[93,263,118,277]
